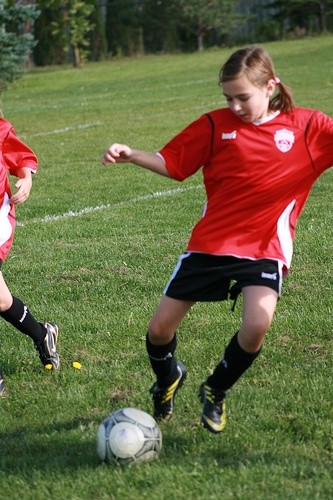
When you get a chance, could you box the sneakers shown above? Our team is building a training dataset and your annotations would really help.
[0,374,6,396]
[149,362,187,421]
[200,382,226,433]
[35,321,60,370]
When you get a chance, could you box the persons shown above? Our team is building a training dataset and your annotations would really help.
[0,111,59,397]
[101,47,333,433]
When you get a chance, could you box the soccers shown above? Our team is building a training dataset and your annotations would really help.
[96,407,164,468]
